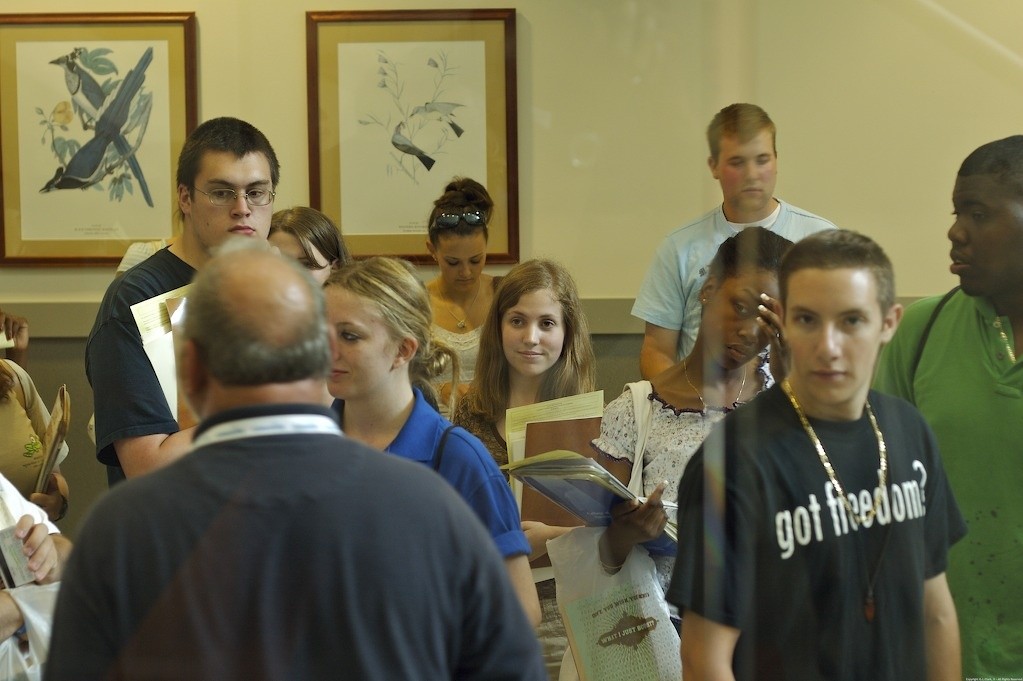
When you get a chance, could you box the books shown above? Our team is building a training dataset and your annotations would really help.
[507,457,678,557]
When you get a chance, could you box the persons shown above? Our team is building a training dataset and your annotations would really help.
[0,102,1023,681]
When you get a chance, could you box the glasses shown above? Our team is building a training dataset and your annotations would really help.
[186,185,276,206]
[429,212,485,233]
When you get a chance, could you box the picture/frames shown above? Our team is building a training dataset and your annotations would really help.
[304,7,521,265]
[1,12,197,269]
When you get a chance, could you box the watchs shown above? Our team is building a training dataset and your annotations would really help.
[55,491,69,522]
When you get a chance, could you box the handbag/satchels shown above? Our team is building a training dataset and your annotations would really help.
[546,527,683,681]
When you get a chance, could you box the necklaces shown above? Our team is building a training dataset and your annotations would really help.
[992,312,1016,365]
[684,356,747,409]
[781,377,886,524]
[436,272,481,329]
[858,496,893,622]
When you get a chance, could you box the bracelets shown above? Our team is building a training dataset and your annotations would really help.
[13,626,26,638]
[600,560,624,571]
